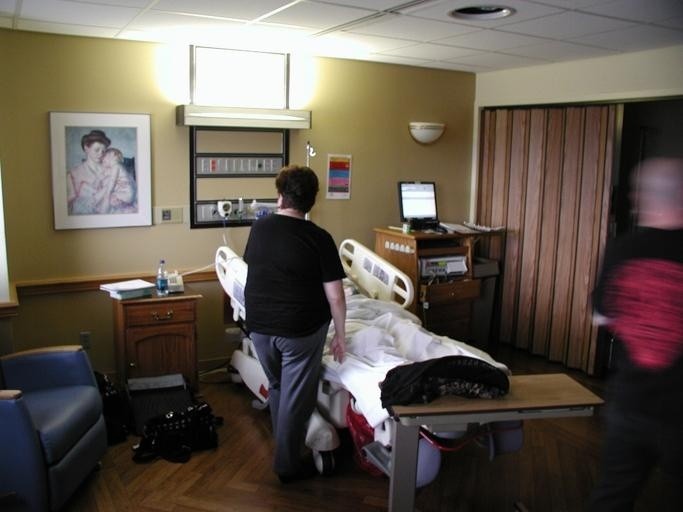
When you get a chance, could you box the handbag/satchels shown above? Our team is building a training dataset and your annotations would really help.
[380,356,508,407]
[97,372,131,436]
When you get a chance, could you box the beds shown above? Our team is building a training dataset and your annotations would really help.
[216,238,512,478]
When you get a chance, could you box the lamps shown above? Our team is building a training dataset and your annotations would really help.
[408,120,446,144]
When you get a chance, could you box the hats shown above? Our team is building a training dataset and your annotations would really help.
[82,131,110,148]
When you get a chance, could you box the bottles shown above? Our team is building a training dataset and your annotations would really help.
[156,260,169,297]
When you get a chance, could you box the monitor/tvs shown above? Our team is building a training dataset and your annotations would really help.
[398,181,438,230]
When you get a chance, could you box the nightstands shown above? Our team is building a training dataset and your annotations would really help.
[113,284,203,396]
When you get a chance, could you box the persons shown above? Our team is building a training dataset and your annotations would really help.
[581,148,682,510]
[242,164,347,488]
[68,130,139,215]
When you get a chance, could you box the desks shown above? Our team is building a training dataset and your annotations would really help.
[388,373,605,511]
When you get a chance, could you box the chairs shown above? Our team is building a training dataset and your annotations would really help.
[0,344,109,511]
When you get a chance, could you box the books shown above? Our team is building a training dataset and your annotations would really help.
[99,278,157,302]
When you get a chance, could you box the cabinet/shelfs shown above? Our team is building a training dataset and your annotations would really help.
[373,224,481,345]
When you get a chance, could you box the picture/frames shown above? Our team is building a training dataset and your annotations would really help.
[46,110,153,232]
